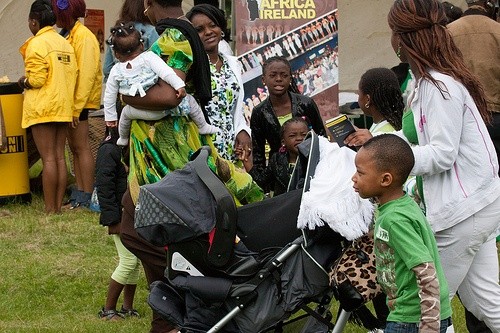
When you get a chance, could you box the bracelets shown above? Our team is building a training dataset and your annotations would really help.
[24,78,28,88]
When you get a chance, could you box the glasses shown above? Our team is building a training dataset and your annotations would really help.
[144,5,150,16]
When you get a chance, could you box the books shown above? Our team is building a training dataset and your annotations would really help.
[324,114,357,147]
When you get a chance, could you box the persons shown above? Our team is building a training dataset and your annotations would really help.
[186,4,252,171]
[18,0,80,215]
[352,133,453,333]
[343,0,500,333]
[240,10,338,122]
[235,117,310,197]
[91,0,160,213]
[120,0,212,333]
[357,67,405,333]
[52,0,102,211]
[95,97,141,322]
[444,5,500,163]
[104,22,222,145]
[250,56,328,173]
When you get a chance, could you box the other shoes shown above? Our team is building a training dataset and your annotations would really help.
[120,305,141,318]
[62,188,91,212]
[98,307,124,321]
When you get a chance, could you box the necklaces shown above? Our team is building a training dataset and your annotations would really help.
[370,118,384,133]
[177,15,185,19]
[213,56,219,67]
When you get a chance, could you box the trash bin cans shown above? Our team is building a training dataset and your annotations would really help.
[0,77,32,205]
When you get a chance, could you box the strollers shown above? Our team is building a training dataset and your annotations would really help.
[132,130,353,333]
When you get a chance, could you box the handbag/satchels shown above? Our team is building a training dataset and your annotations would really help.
[329,234,383,310]
